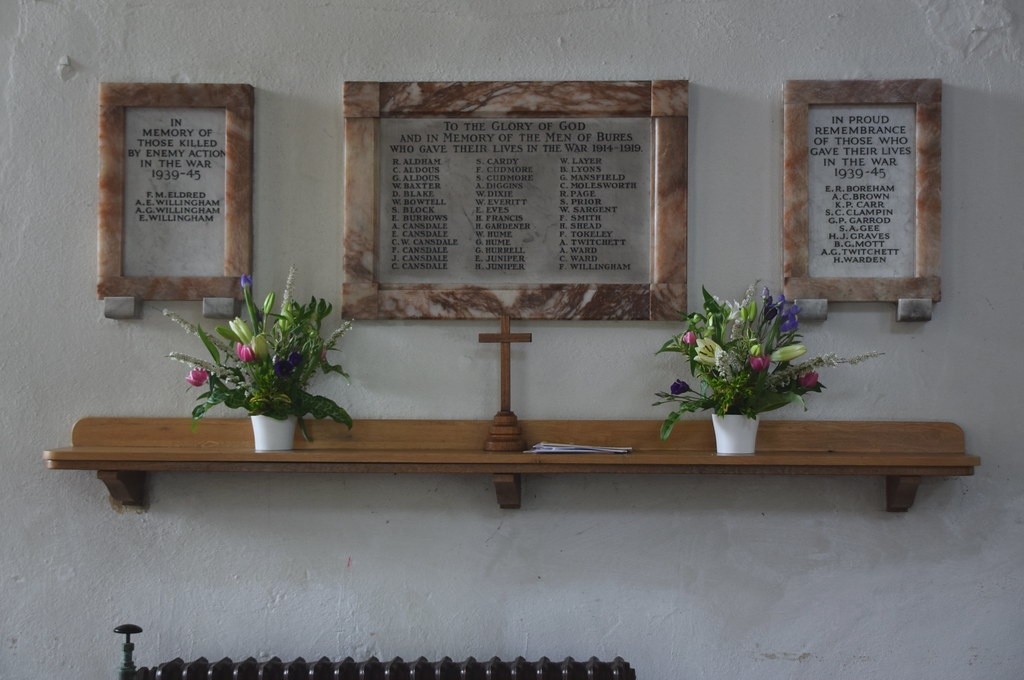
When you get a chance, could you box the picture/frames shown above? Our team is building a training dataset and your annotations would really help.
[781,79,944,305]
[97,83,250,300]
[338,78,692,321]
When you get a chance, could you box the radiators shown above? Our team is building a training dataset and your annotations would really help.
[112,623,639,680]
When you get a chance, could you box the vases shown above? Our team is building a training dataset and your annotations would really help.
[249,413,294,455]
[712,414,757,457]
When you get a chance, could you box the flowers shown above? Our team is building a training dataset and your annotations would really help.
[161,265,355,443]
[646,281,883,439]
